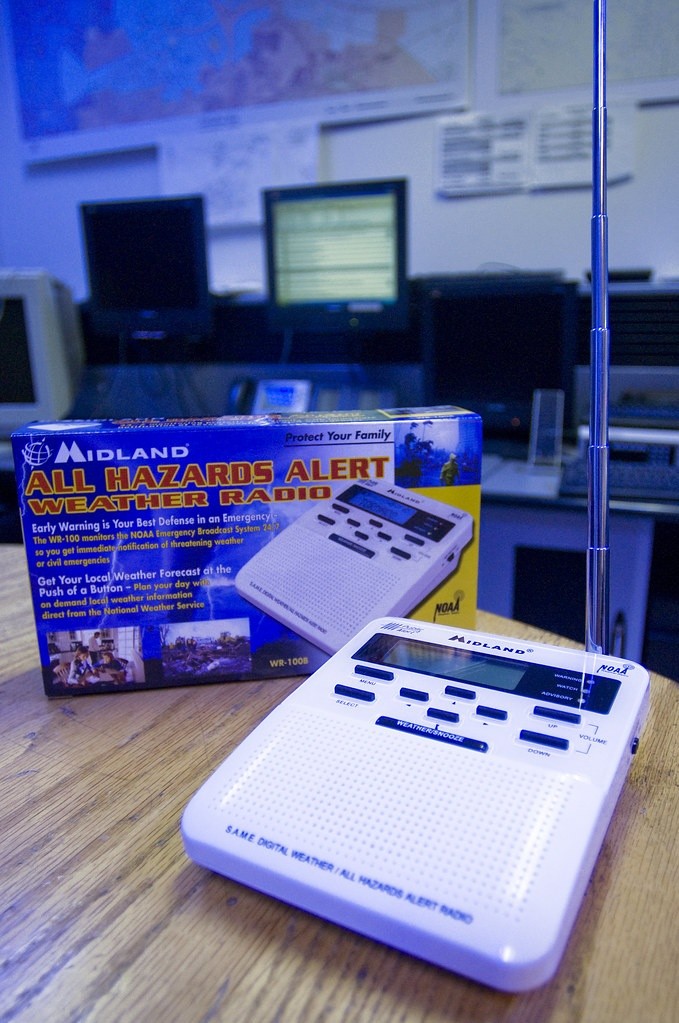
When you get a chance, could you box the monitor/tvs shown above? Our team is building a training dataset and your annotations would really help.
[264,178,410,338]
[421,281,578,446]
[78,196,213,337]
[0,266,84,440]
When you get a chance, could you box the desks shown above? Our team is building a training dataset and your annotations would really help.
[0,542,679,1023]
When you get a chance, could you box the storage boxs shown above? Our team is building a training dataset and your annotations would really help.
[10,403,484,700]
